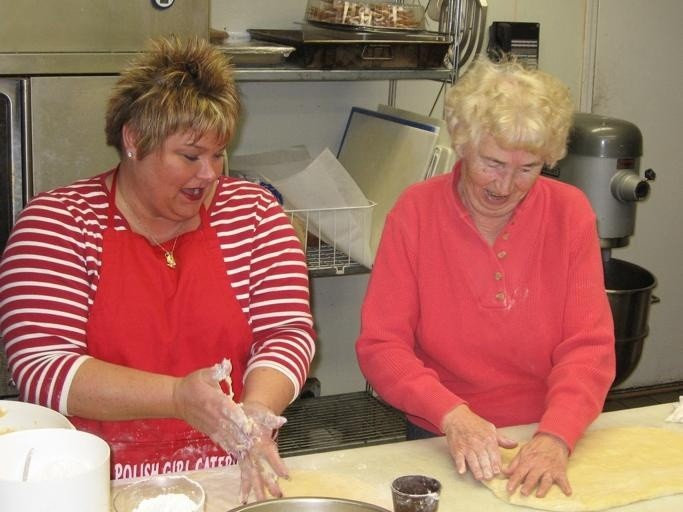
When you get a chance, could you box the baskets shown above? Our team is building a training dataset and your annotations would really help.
[281,200,378,276]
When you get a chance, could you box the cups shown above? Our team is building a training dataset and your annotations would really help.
[112,476,205,512]
[392,475,442,511]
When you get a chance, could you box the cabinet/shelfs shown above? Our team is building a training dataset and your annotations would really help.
[18,1,466,458]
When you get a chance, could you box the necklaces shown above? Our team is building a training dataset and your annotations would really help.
[146,229,180,268]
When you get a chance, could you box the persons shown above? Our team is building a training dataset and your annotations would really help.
[0,36,317,506]
[355,47,617,498]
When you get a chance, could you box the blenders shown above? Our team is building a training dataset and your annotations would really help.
[557,112,660,391]
